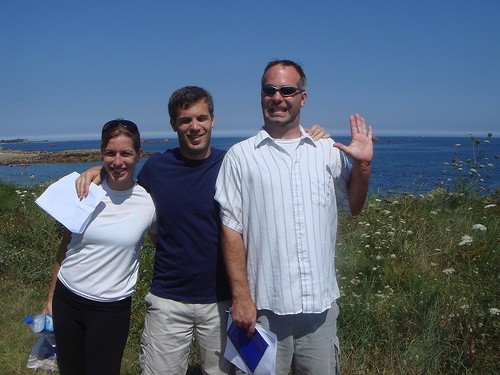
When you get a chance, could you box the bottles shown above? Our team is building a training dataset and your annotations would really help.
[24,314,53,336]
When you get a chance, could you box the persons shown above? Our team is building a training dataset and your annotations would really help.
[40,118,160,375]
[75,86,330,375]
[214,60,373,375]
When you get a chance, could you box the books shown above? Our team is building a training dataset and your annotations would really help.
[227,319,270,374]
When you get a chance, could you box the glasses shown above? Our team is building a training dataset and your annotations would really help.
[102,120,139,135]
[262,86,303,96]
